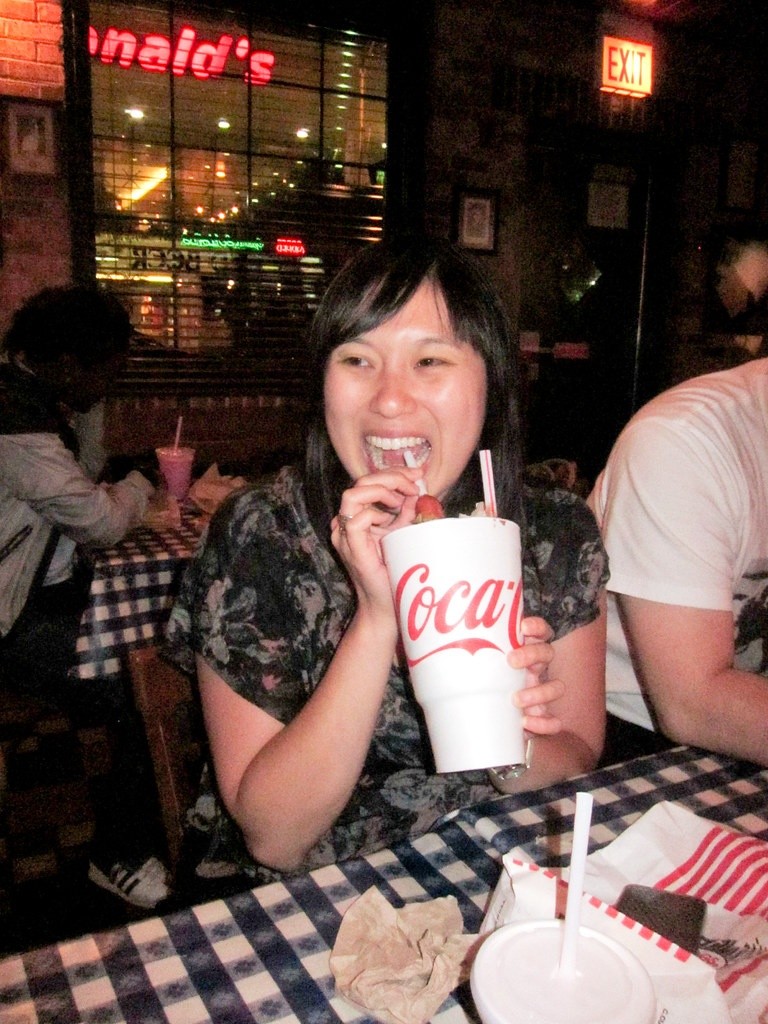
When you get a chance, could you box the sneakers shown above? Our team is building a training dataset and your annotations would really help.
[87,854,176,908]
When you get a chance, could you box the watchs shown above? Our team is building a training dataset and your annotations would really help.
[490,739,533,784]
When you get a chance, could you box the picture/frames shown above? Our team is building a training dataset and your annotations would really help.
[449,183,505,257]
[1,94,65,186]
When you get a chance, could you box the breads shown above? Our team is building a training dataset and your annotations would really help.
[616,883,708,957]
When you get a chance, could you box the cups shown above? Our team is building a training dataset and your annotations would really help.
[378,516,524,774]
[156,447,195,500]
[471,919,657,1024]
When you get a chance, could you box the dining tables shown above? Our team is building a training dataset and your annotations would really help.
[0,742,768,1024]
[64,502,209,680]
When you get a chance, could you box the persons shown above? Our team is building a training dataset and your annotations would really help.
[158,232,610,907]
[586,359,767,772]
[518,225,621,464]
[1,284,177,909]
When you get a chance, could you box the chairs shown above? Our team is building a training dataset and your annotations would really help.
[130,646,242,909]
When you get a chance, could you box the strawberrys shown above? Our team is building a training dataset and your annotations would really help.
[411,494,448,525]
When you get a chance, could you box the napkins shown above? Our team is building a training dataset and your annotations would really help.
[331,885,478,1022]
